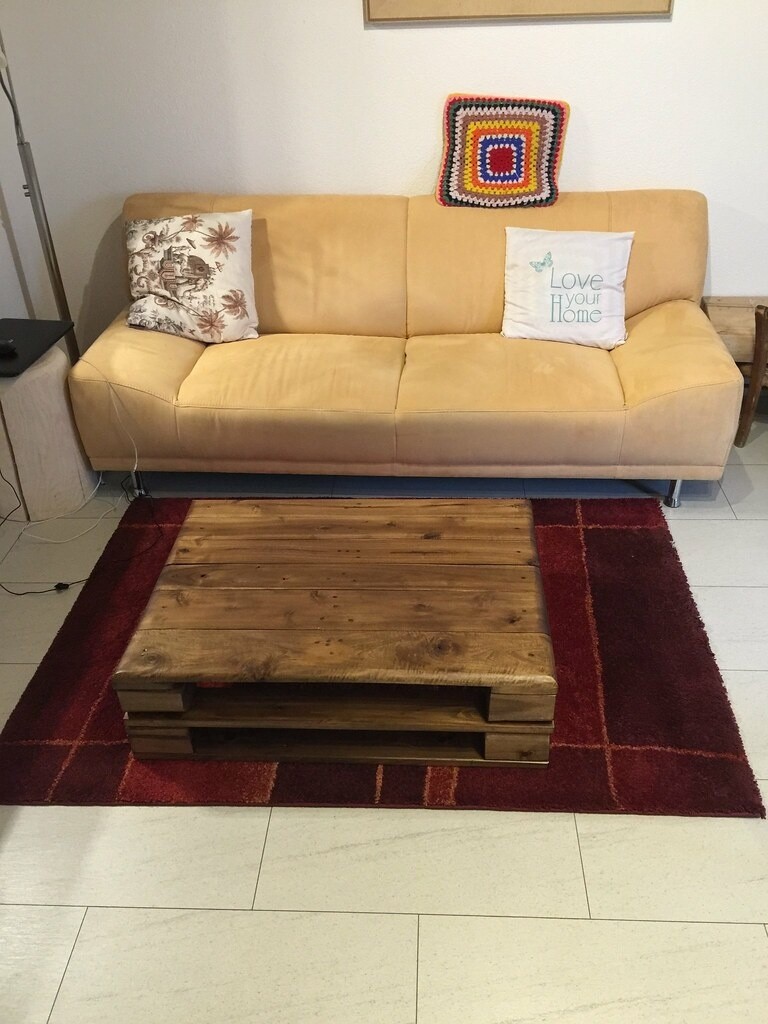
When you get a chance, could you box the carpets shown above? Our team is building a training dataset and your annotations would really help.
[0,497,766,821]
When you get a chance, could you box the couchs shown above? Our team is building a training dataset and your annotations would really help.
[68,191,745,508]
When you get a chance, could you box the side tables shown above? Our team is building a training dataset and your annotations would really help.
[1,329,103,521]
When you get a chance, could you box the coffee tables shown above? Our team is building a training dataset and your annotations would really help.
[112,498,557,767]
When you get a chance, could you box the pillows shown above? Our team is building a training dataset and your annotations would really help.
[501,227,634,350]
[125,209,259,343]
